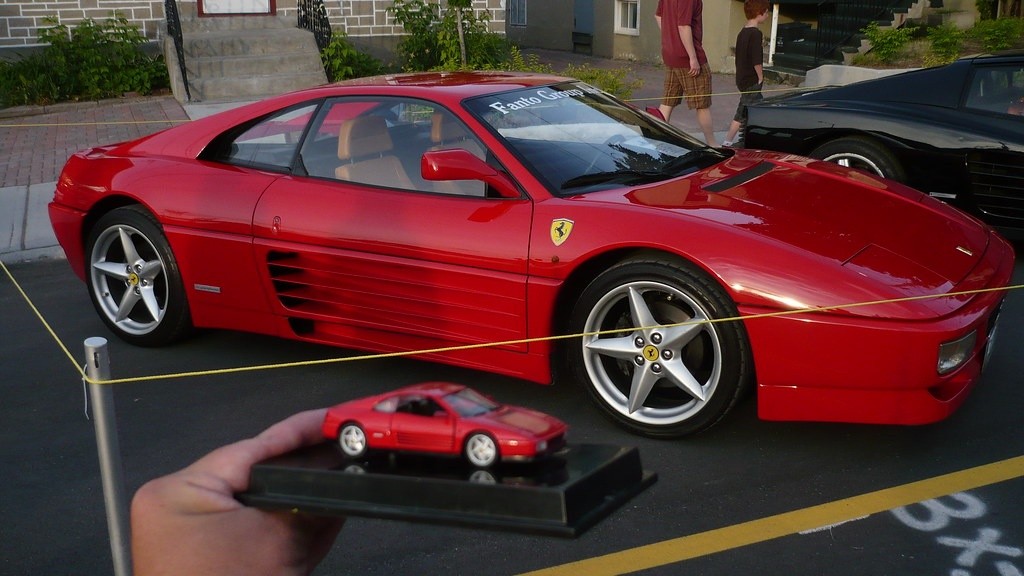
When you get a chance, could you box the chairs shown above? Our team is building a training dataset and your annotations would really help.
[335,115,417,191]
[427,109,486,196]
[966,69,1012,114]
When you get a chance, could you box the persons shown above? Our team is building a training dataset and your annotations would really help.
[132,409,350,576]
[723,0,769,148]
[657,1,723,148]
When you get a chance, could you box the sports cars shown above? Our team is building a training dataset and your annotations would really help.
[739,52,1024,232]
[47,65,1018,441]
[320,380,576,470]
